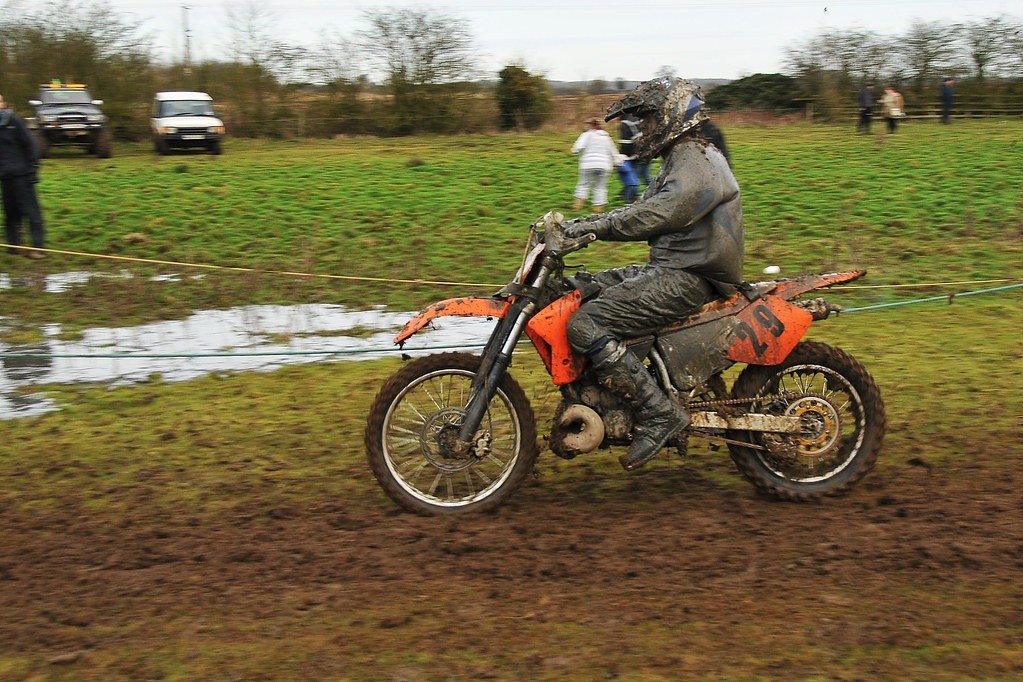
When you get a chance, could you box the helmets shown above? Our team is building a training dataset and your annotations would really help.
[605,77,710,165]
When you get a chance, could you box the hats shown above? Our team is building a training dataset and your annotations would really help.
[584,116,604,125]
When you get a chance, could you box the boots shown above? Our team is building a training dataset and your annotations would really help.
[594,349,690,471]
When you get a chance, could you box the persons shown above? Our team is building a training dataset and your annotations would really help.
[561,77,744,471]
[1,96,45,260]
[856,82,904,134]
[940,78,955,123]
[570,118,653,214]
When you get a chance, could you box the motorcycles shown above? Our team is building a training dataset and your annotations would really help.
[363,209,888,520]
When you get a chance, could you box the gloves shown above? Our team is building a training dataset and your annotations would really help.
[564,219,610,241]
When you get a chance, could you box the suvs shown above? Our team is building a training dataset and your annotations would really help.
[23,80,111,159]
[149,92,225,156]
